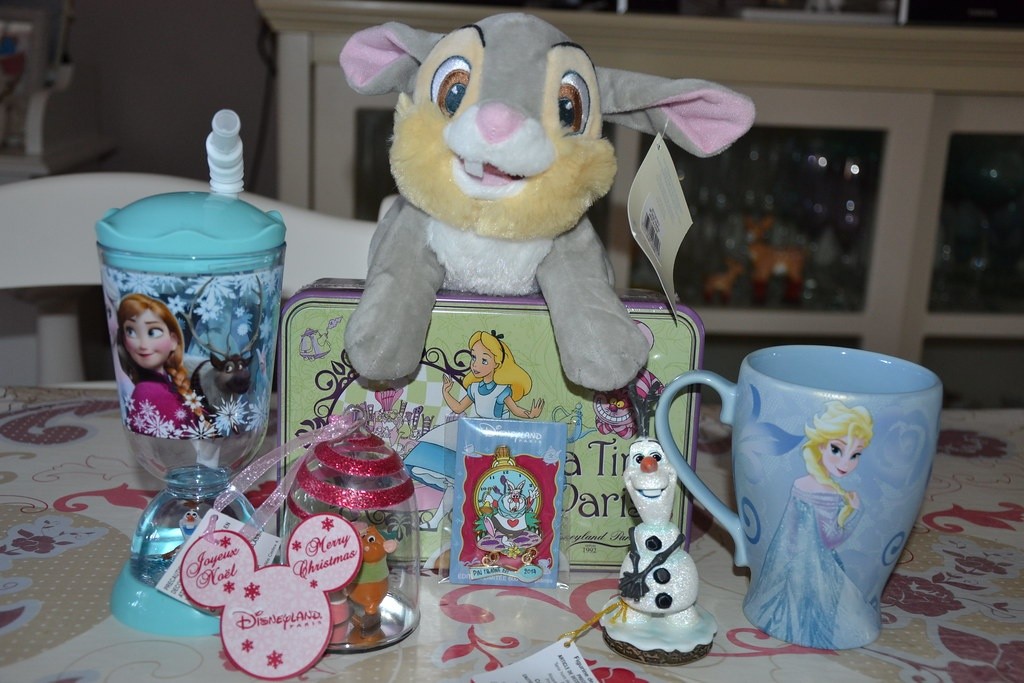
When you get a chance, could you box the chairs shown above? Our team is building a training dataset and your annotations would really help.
[0,173,376,388]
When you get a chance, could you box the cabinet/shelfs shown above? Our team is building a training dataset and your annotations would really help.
[251,0,1024,408]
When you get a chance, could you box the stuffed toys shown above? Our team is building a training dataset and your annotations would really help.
[339,11,757,392]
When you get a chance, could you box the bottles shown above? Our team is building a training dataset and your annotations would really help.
[95,191,288,636]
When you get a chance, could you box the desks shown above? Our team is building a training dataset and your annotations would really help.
[0,383,1024,683]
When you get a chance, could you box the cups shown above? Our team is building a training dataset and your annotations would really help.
[653,344,943,650]
